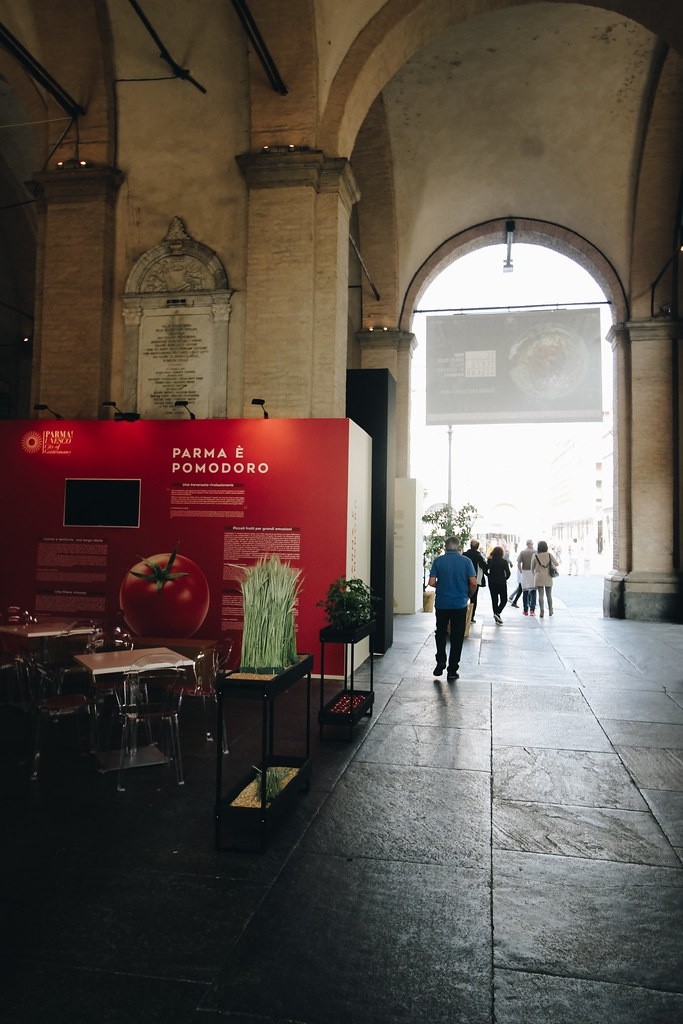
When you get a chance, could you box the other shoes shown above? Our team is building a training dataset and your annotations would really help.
[433,661,446,676]
[530,610,535,615]
[447,672,460,679]
[522,610,528,615]
[508,597,513,603]
[470,619,476,624]
[494,614,503,624]
[511,603,519,608]
[549,612,554,616]
[540,613,543,617]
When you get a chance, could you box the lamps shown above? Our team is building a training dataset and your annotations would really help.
[33,403,64,420]
[174,400,196,420]
[101,401,126,420]
[251,399,269,420]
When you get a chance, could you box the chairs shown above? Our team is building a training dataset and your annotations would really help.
[0,604,234,793]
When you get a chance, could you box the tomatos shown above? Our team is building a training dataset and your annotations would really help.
[120,541,209,639]
[330,695,365,714]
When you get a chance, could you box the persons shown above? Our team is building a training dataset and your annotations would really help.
[567,539,582,577]
[487,547,511,623]
[428,536,477,679]
[509,540,559,618]
[462,540,487,622]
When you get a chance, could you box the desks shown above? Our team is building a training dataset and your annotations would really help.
[0,622,97,754]
[73,645,197,773]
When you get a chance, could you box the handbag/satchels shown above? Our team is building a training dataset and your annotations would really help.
[549,564,559,577]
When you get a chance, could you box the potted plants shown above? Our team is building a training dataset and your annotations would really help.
[422,501,483,638]
[211,552,382,853]
